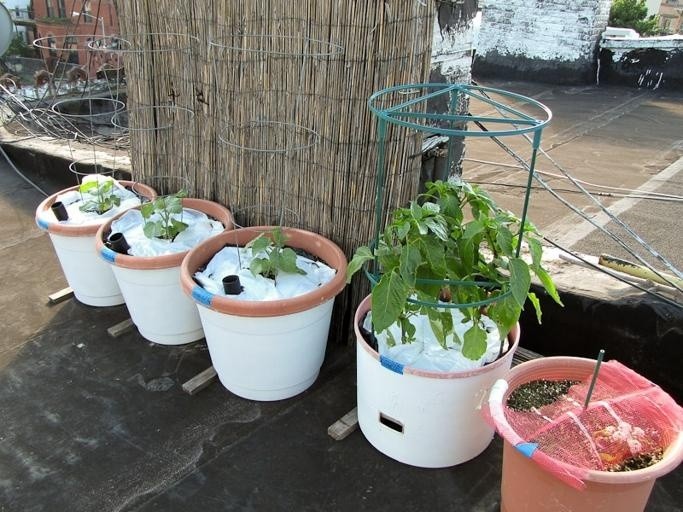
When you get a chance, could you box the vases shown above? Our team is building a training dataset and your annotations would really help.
[487,355,683,512]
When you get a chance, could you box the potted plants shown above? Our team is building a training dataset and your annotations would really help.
[35,180,159,306]
[345,176,564,470]
[181,225,348,401]
[95,198,234,346]
